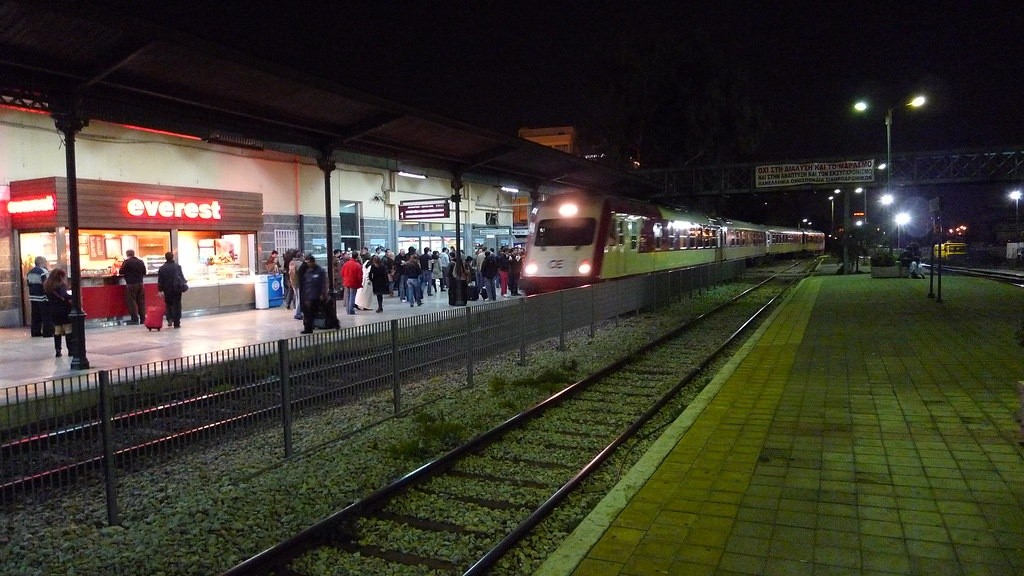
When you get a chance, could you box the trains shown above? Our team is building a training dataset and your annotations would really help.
[517,193,826,297]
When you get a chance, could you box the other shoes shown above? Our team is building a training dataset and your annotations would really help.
[126,319,140,325]
[174,323,181,328]
[428,293,433,296]
[440,289,446,292]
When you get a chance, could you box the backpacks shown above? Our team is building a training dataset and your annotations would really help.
[486,256,496,274]
[449,259,463,278]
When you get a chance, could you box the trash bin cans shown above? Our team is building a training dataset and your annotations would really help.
[901,258,910,278]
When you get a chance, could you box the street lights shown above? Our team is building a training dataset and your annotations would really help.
[829,195,834,250]
[854,94,937,249]
[891,209,911,250]
[1009,189,1022,226]
[854,188,867,248]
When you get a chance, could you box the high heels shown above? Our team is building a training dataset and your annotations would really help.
[376,307,384,313]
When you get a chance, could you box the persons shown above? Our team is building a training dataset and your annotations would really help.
[157,252,186,328]
[608,233,615,246]
[26,256,54,337]
[110,259,124,275]
[334,245,526,314]
[266,250,281,273]
[900,238,925,279]
[119,250,147,325]
[44,269,74,357]
[283,249,341,334]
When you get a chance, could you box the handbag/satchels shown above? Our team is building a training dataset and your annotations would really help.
[382,270,391,283]
[173,262,188,293]
[293,267,300,289]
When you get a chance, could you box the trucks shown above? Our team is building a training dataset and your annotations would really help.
[933,241,970,260]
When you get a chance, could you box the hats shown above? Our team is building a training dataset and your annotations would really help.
[409,247,416,250]
[465,256,473,261]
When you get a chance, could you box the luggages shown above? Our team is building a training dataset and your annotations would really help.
[313,292,341,330]
[466,281,479,301]
[144,294,166,332]
[480,282,488,301]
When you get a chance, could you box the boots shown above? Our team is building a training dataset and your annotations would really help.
[65,333,73,356]
[53,334,63,357]
